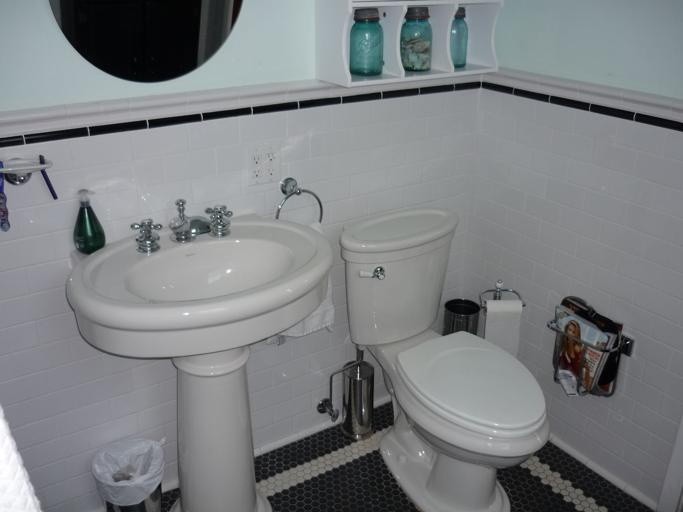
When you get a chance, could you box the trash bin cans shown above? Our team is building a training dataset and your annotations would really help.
[90,436,166,511]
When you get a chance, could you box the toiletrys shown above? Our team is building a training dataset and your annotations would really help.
[72,189,106,254]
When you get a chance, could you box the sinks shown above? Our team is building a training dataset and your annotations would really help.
[66,212,334,357]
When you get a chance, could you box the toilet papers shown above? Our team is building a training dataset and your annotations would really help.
[482,298,523,360]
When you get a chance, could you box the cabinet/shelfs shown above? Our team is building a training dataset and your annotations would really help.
[313,0,503,89]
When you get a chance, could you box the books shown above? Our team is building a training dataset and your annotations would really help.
[552,303,617,392]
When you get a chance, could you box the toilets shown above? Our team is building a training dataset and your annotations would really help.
[340,206,552,512]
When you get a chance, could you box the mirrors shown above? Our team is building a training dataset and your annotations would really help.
[47,1,249,83]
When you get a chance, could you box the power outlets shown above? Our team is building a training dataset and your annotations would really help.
[249,149,280,189]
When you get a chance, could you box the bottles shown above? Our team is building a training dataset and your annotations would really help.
[350,7,469,74]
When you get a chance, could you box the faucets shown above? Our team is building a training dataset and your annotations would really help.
[167,198,212,244]
